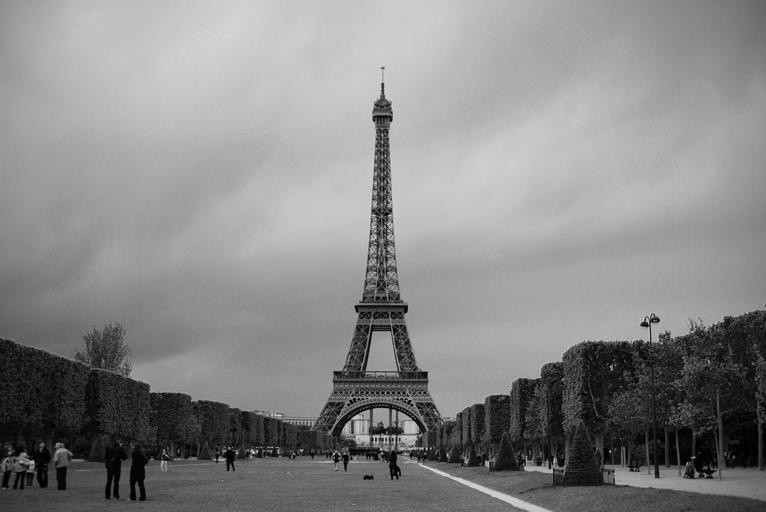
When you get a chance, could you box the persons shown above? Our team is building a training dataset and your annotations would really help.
[388,450,400,480]
[341,450,349,472]
[222,446,236,472]
[53,441,73,489]
[332,449,341,471]
[34,439,50,488]
[103,434,128,500]
[128,441,148,501]
[0,440,36,489]
[160,448,169,472]
[287,446,428,464]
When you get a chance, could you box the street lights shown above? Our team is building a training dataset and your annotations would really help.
[636,312,661,480]
